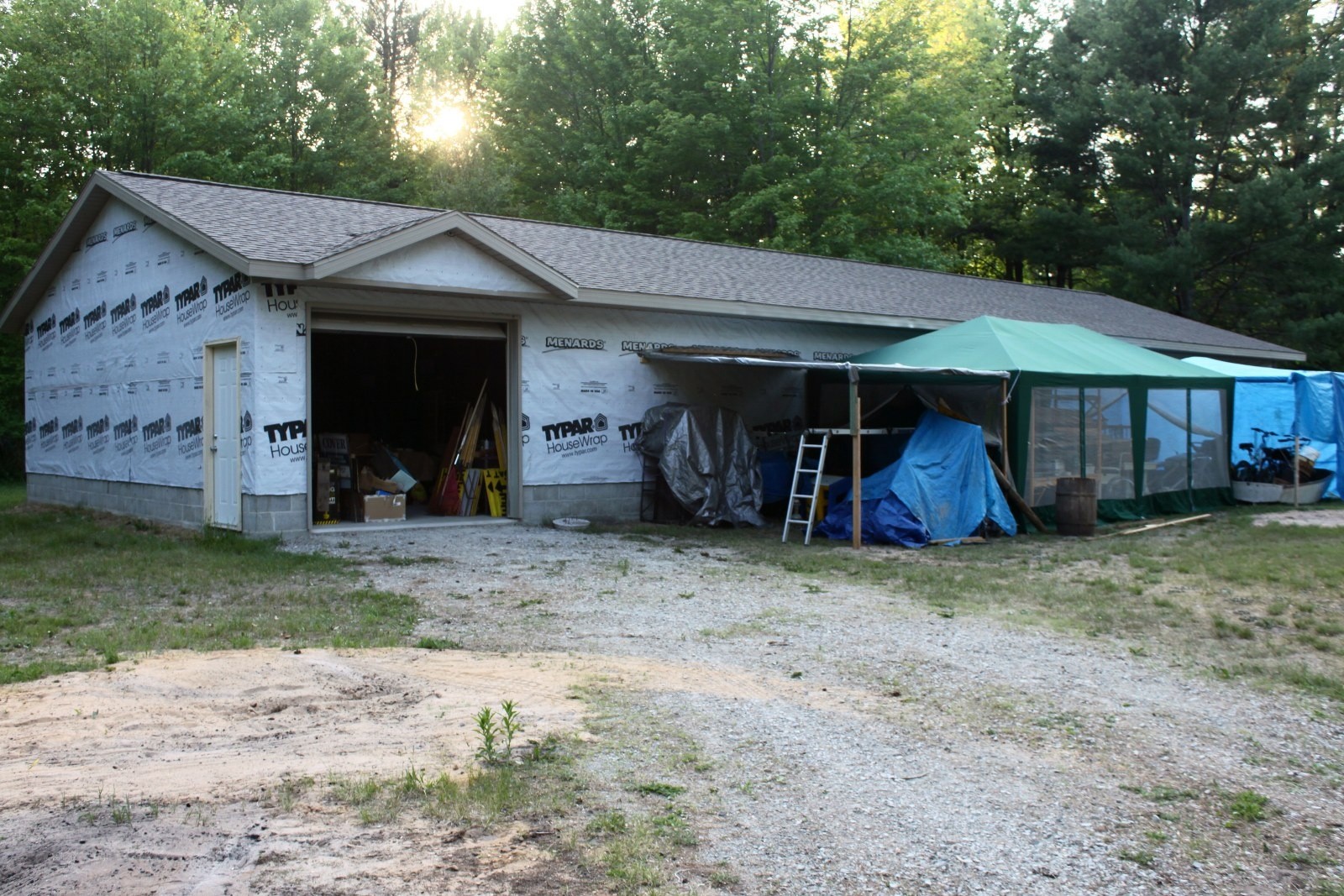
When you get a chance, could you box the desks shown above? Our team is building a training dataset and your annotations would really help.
[395,450,438,482]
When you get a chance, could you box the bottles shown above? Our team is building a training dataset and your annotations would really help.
[361,467,367,480]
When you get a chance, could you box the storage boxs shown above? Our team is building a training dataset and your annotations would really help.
[347,493,407,522]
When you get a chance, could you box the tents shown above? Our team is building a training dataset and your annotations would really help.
[1121,356,1344,502]
[809,315,1234,532]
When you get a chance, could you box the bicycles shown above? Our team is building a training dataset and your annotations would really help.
[1238,427,1314,482]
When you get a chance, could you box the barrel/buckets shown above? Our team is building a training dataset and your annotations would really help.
[1056,477,1097,536]
[1124,462,1157,496]
[1301,446,1320,462]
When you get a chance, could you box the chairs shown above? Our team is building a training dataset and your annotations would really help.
[805,473,845,522]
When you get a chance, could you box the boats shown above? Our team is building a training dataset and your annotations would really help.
[1233,468,1334,505]
